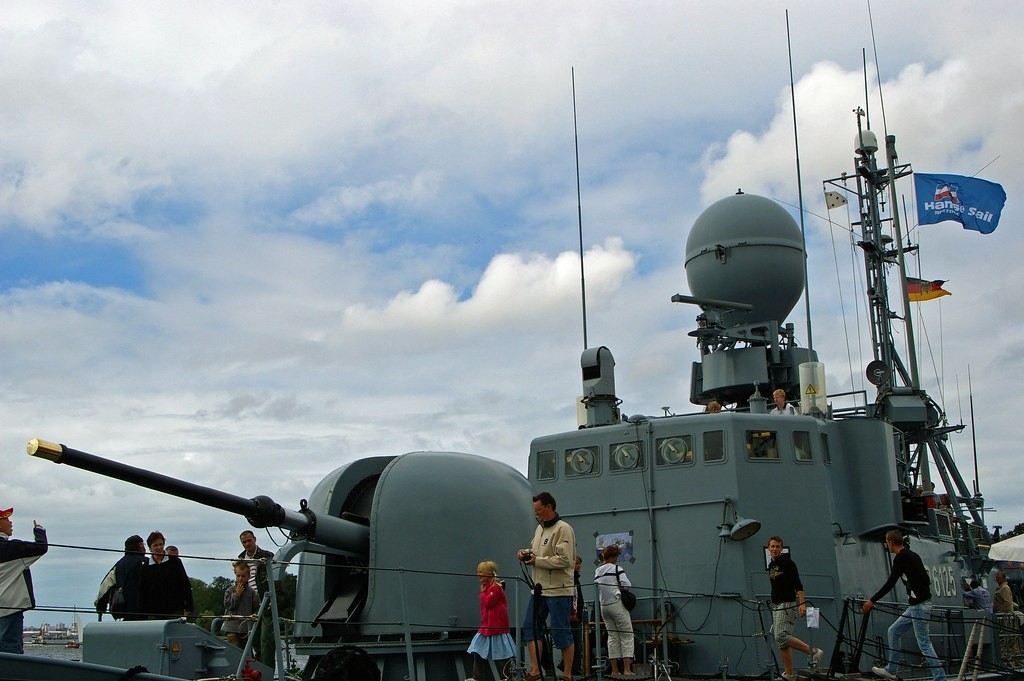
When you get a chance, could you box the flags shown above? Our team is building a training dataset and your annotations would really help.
[913,172,1007,235]
[904,276,952,302]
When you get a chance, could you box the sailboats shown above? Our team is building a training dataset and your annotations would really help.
[64,603,83,649]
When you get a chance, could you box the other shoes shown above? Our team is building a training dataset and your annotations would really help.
[521,672,541,681]
[559,676,571,681]
[808,647,824,672]
[774,672,795,681]
[871,666,896,680]
[465,678,477,681]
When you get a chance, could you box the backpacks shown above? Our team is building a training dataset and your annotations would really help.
[302,645,382,681]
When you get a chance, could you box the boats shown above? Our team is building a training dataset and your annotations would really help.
[29,631,79,645]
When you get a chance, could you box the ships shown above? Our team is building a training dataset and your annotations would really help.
[0,1,1024,681]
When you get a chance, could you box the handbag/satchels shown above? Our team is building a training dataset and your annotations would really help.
[620,588,637,612]
[107,586,128,621]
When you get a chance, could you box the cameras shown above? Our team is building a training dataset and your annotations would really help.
[531,583,542,598]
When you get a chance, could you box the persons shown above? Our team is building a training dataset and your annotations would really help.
[595,545,635,676]
[708,401,721,414]
[861,530,948,681]
[95,531,193,621]
[768,536,824,681]
[464,561,511,681]
[770,389,798,416]
[963,580,992,671]
[224,563,261,655]
[237,530,276,679]
[0,508,49,653]
[556,556,585,676]
[517,492,576,681]
[917,482,935,495]
[993,571,1013,614]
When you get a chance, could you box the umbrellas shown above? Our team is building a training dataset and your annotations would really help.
[988,533,1024,563]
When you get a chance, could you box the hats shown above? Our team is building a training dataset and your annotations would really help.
[0,508,14,519]
